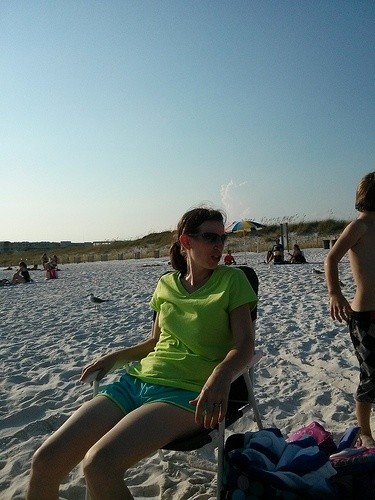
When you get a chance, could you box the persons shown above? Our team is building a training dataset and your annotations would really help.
[223,250,236,265]
[288,244,302,263]
[12,261,30,285]
[323,171,375,449]
[26,207,260,500]
[7,253,57,270]
[265,239,284,264]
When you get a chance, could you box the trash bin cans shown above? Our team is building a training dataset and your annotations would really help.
[323,240,330,249]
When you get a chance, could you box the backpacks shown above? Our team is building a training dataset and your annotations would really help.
[295,254,306,263]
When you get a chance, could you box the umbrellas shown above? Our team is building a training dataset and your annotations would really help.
[224,218,267,265]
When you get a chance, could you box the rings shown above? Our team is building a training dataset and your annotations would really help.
[214,403,220,407]
[102,368,109,373]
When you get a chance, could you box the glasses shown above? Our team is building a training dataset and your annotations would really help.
[182,232,224,245]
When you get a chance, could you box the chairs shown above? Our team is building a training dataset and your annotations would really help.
[78,265,264,500]
[264,244,284,266]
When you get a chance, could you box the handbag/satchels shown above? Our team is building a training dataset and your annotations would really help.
[285,420,337,460]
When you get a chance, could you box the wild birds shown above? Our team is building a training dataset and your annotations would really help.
[87,293,114,310]
